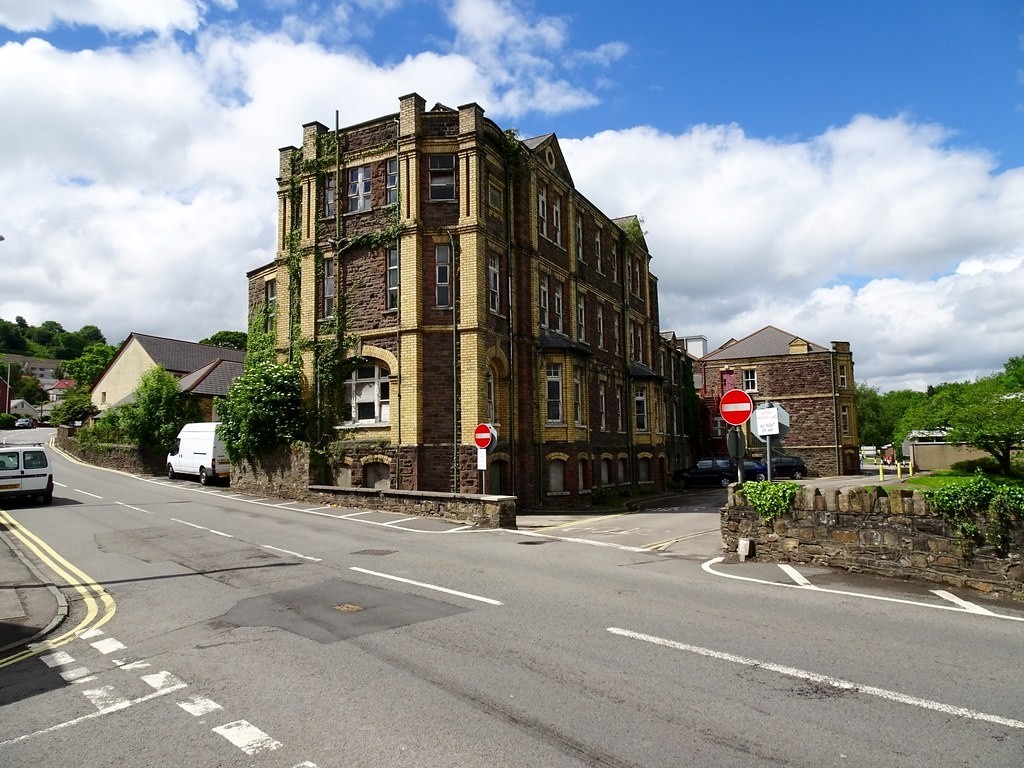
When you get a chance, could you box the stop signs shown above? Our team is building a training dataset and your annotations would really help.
[718,389,752,426]
[474,424,492,450]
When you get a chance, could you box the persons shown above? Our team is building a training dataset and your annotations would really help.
[859,452,864,467]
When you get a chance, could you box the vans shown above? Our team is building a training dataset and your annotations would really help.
[165,421,236,487]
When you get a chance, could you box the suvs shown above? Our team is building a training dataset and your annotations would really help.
[672,456,746,488]
[758,456,809,480]
[0,441,54,507]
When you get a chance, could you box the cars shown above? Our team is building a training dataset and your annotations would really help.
[15,418,32,429]
[30,418,38,429]
[732,458,775,483]
